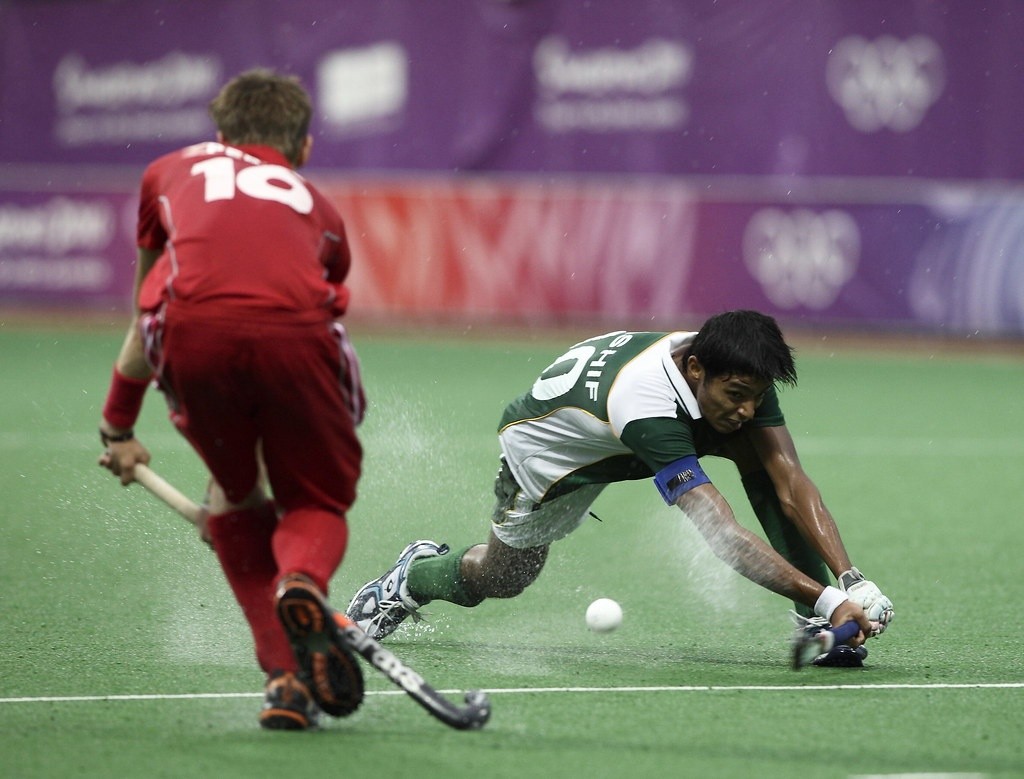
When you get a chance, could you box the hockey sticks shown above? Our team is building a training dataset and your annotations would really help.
[786,621,864,674]
[126,456,495,733]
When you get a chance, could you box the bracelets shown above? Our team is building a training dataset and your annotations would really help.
[99,427,135,445]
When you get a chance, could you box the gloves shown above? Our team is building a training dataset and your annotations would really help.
[839,565,893,628]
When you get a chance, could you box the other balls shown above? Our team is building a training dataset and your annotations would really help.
[582,595,625,635]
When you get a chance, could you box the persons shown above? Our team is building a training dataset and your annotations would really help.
[346,308,898,667]
[95,67,369,729]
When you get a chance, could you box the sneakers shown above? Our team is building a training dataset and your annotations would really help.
[259,671,321,731]
[273,571,364,717]
[344,540,450,643]
[787,607,866,668]
